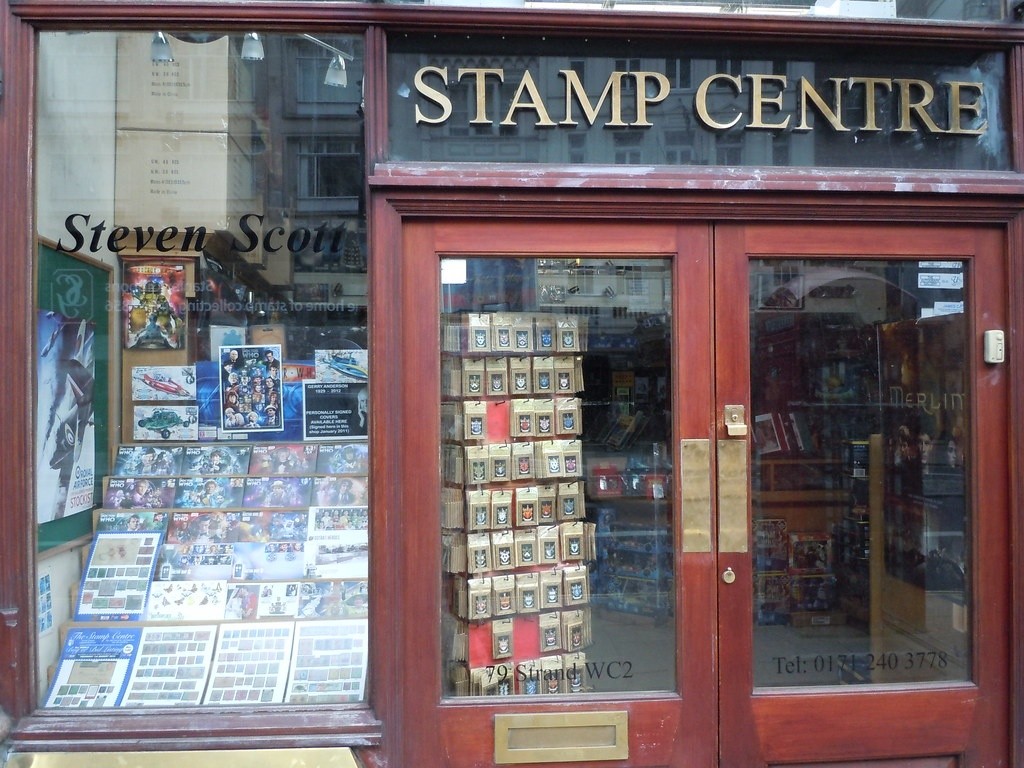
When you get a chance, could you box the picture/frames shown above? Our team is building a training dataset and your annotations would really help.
[757,272,806,309]
[38,234,117,560]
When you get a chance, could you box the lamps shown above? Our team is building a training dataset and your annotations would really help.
[151,31,174,62]
[324,54,347,88]
[240,31,265,60]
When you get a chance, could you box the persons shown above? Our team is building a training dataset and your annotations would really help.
[883,426,966,590]
[109,444,369,618]
[348,385,370,436]
[221,349,283,427]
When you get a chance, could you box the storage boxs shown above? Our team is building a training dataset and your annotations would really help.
[593,463,672,621]
[753,519,849,625]
[116,34,294,290]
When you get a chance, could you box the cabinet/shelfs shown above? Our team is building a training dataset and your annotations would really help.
[113,230,287,446]
